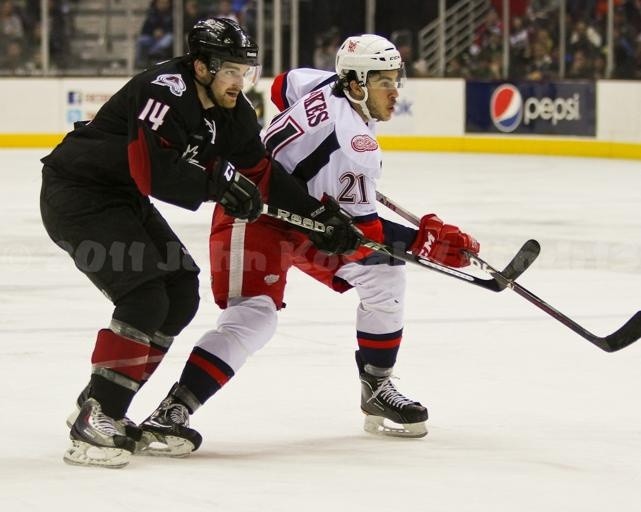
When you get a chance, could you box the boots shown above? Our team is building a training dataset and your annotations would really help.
[79,375,144,443]
[138,380,204,451]
[354,348,430,425]
[68,389,140,455]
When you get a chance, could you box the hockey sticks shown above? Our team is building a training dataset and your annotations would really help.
[261,204,540,292]
[376,190,640,352]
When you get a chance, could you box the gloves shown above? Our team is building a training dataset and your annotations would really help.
[407,211,483,268]
[299,196,365,258]
[204,153,265,225]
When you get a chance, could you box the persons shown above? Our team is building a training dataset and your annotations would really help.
[135,1,641,80]
[138,34,480,451]
[0,0,78,71]
[38,16,365,452]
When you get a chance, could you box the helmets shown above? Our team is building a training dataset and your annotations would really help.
[333,32,403,85]
[187,16,262,71]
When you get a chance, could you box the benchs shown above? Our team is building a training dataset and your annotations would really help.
[0,0,289,81]
